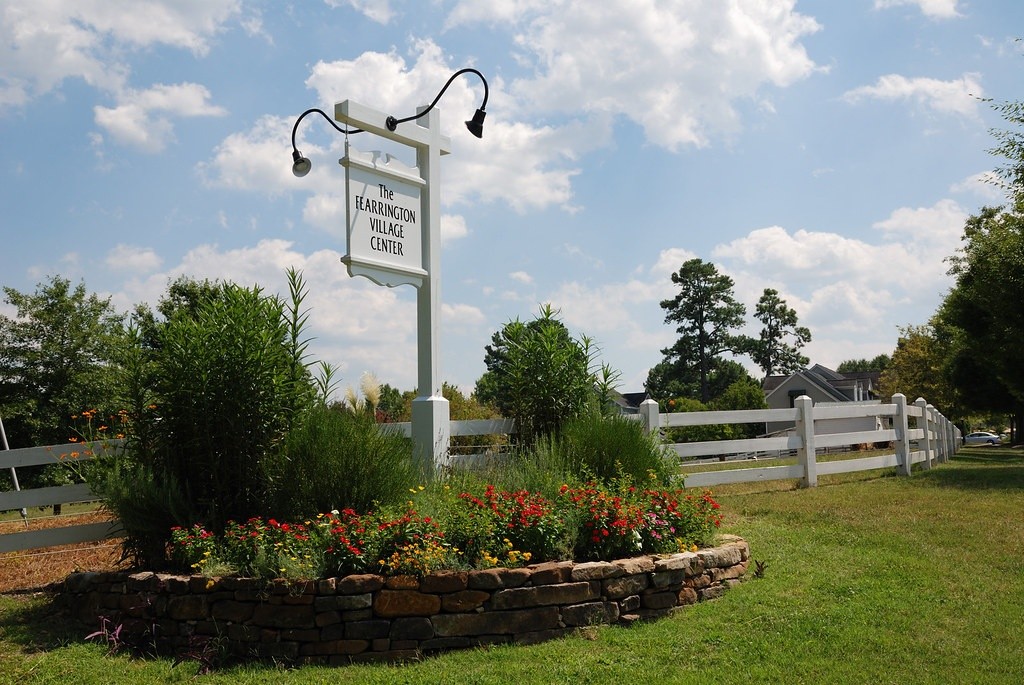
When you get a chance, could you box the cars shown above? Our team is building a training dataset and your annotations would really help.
[963,432,1001,445]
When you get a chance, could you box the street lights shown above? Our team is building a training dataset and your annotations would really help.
[290,66,488,466]
